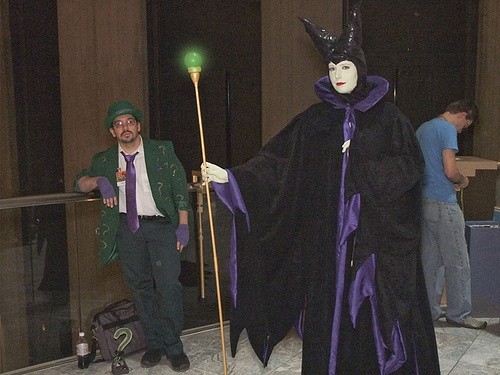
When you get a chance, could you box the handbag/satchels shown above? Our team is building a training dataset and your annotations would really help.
[92,299,147,362]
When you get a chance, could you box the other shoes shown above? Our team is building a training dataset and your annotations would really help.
[164,346,189,371]
[446,315,487,329]
[434,314,447,325]
[141,348,162,368]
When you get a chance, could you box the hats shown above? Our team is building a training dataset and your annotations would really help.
[104,100,142,130]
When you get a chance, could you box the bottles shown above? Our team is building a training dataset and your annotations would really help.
[76,331,90,369]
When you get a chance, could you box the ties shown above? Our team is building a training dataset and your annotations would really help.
[121,151,139,233]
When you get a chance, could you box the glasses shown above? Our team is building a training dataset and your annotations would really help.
[113,119,135,127]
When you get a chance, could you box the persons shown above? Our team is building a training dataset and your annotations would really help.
[200,1,441,375]
[408,99,488,330]
[74,101,197,372]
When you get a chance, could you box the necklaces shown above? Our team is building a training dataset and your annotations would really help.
[439,116,448,121]
